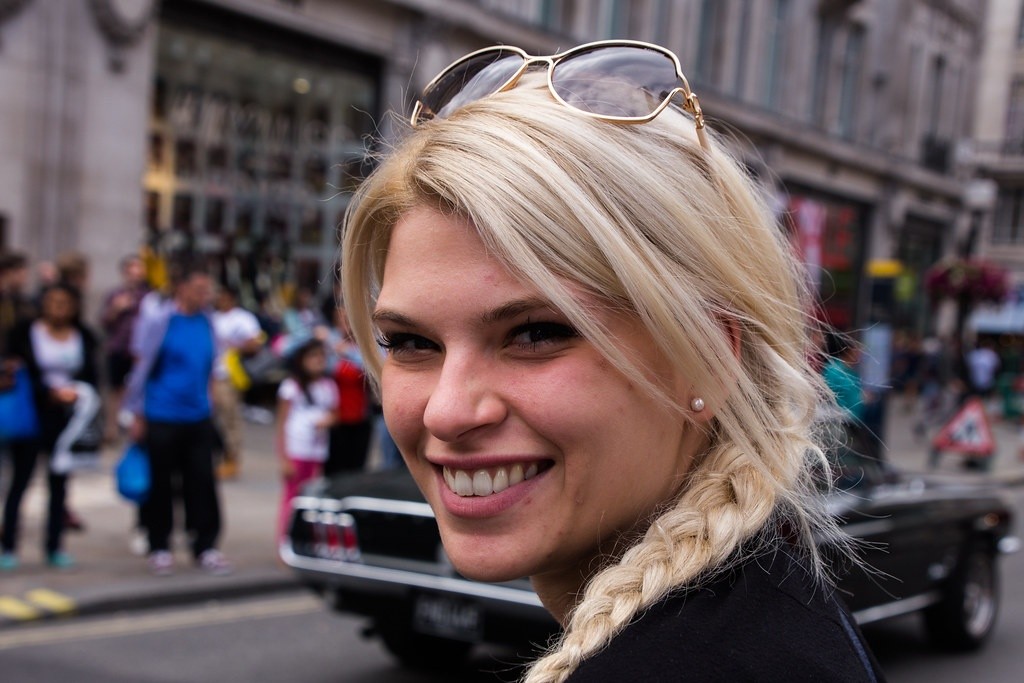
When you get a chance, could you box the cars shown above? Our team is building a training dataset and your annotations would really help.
[282,409,1023,683]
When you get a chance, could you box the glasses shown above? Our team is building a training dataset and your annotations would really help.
[409,38,714,161]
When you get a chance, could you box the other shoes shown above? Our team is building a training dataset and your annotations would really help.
[48,554,73,568]
[148,548,172,576]
[126,527,150,557]
[0,550,16,569]
[197,548,235,577]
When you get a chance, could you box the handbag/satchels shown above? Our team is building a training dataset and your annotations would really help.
[0,372,39,443]
[222,349,253,396]
[117,445,151,503]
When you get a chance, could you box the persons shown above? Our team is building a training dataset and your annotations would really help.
[0,248,402,578]
[805,324,1001,473]
[338,38,886,683]
